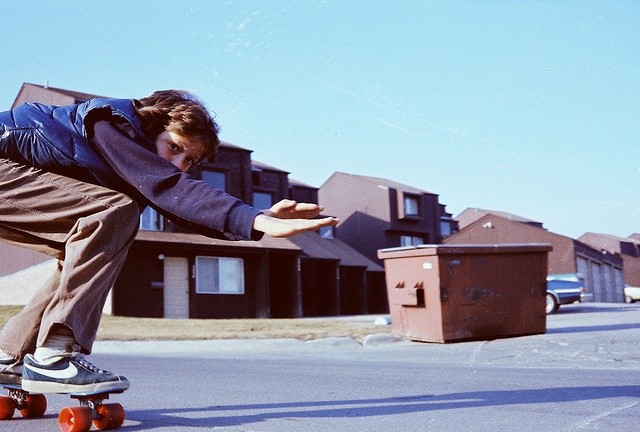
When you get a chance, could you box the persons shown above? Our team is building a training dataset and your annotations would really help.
[0,88,340,395]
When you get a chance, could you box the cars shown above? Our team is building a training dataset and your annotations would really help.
[624,285,640,303]
[546,273,585,314]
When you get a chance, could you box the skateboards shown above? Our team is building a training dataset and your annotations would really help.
[0,383,125,432]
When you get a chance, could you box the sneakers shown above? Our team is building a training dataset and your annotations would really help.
[0,359,22,384]
[22,345,130,393]
